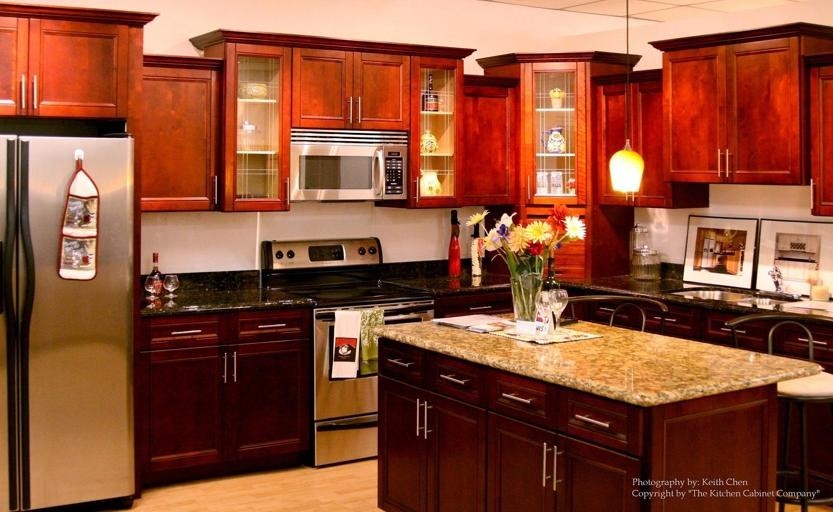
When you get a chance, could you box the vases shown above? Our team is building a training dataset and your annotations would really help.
[509,269,543,334]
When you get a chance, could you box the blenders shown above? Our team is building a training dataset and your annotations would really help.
[630,224,660,280]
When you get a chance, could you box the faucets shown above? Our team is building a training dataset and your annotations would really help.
[769,267,783,296]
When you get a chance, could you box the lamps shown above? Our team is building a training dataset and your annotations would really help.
[608,138,647,204]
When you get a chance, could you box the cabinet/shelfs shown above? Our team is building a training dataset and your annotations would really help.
[463,74,519,205]
[137,54,222,214]
[648,22,833,185]
[140,275,318,487]
[800,50,833,219]
[0,3,160,120]
[372,312,821,512]
[590,69,710,209]
[475,50,644,286]
[383,270,510,318]
[189,29,478,213]
[383,276,833,507]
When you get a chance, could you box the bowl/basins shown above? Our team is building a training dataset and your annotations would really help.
[238,83,268,99]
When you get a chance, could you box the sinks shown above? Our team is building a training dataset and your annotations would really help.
[737,297,789,309]
[671,289,754,302]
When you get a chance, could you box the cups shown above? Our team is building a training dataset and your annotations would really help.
[550,171,563,196]
[144,276,161,300]
[545,290,569,337]
[536,171,548,195]
[163,275,180,298]
[534,290,554,336]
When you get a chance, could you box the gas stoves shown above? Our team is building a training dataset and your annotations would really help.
[261,237,434,307]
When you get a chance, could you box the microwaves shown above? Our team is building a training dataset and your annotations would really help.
[289,127,408,201]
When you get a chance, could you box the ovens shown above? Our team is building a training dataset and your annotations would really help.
[311,301,435,467]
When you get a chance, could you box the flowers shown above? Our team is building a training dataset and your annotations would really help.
[464,206,586,334]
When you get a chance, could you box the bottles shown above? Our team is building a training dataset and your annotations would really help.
[143,251,163,297]
[422,75,438,112]
[543,247,561,290]
[448,210,460,278]
[470,223,483,276]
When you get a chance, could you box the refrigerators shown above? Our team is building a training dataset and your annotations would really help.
[1,134,135,512]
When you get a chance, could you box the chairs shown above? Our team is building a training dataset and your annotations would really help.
[567,290,668,332]
[724,310,833,512]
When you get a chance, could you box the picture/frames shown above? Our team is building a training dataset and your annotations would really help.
[755,218,833,302]
[681,213,758,294]
